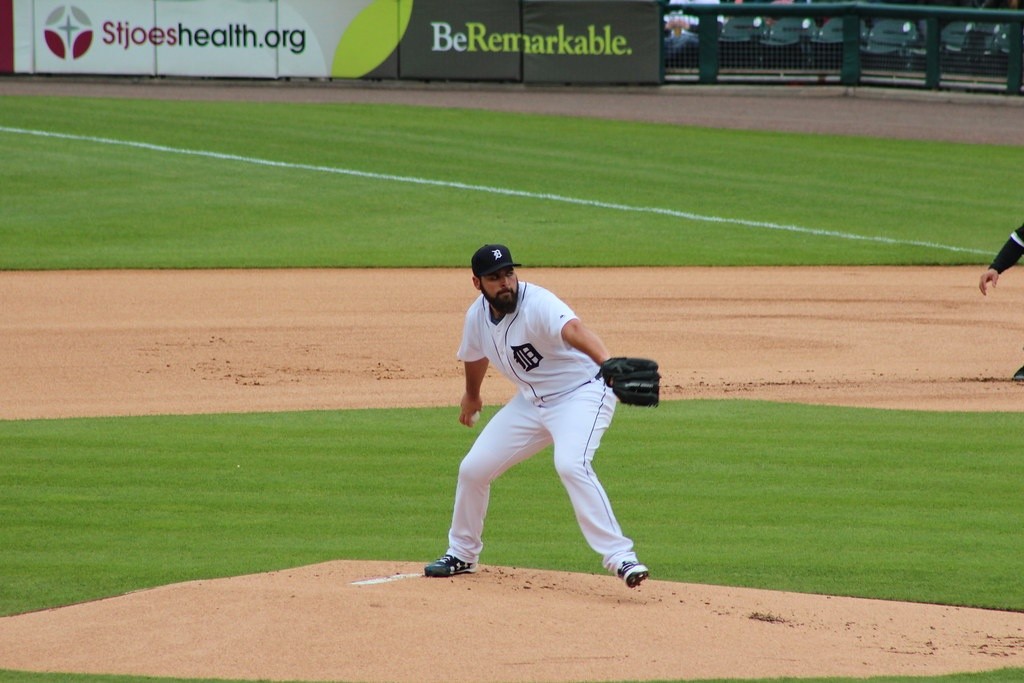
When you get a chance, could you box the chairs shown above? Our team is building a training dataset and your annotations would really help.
[718,16,1011,77]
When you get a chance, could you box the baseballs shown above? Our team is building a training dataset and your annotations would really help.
[471,410,480,423]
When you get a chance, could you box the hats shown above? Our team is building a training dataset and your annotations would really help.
[471,244,522,275]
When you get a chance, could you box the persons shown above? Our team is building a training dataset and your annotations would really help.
[979,224,1024,378]
[424,244,661,588]
[663,0,725,68]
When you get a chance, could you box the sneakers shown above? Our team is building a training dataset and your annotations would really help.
[425,554,477,578]
[617,560,649,589]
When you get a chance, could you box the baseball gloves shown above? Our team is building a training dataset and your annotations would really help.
[595,356,662,409]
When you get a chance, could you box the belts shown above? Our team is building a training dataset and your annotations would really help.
[584,368,603,385]
